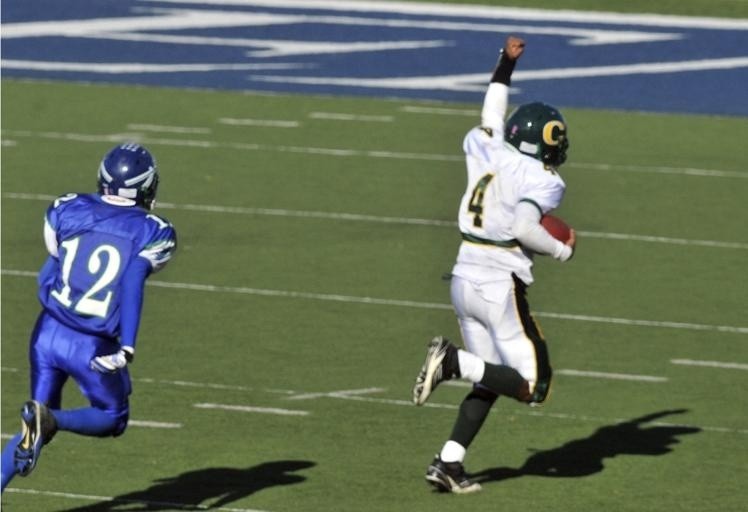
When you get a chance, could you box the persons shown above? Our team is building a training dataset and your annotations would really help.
[2,140,183,495]
[409,35,578,495]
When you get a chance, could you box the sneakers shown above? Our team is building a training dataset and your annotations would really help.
[423,451,482,495]
[412,334,456,408]
[13,399,51,478]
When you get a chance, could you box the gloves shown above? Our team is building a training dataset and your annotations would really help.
[89,344,136,376]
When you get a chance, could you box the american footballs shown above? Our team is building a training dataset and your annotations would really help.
[517,213,575,256]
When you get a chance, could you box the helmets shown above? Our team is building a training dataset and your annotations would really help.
[95,142,160,212]
[503,100,571,168]
